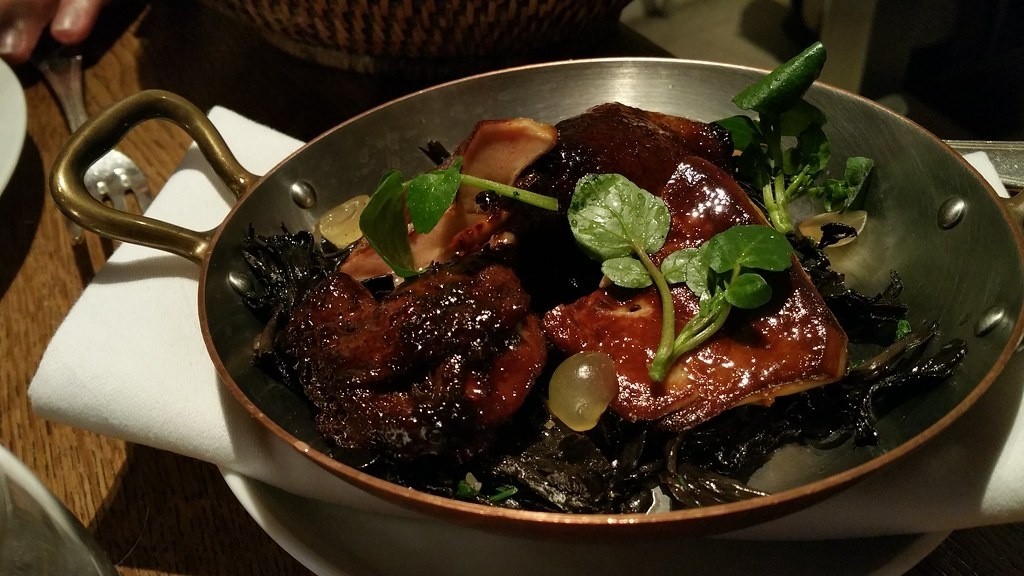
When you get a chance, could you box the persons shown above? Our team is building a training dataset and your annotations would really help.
[0,0,106,66]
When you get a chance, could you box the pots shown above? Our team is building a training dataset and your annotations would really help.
[46,54,1023,543]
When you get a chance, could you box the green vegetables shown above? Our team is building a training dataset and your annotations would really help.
[360,39,875,383]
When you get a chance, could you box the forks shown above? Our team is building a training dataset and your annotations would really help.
[31,29,152,244]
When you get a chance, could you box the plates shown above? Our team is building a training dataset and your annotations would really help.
[0,58,28,197]
[215,465,953,575]
[1,445,118,576]
